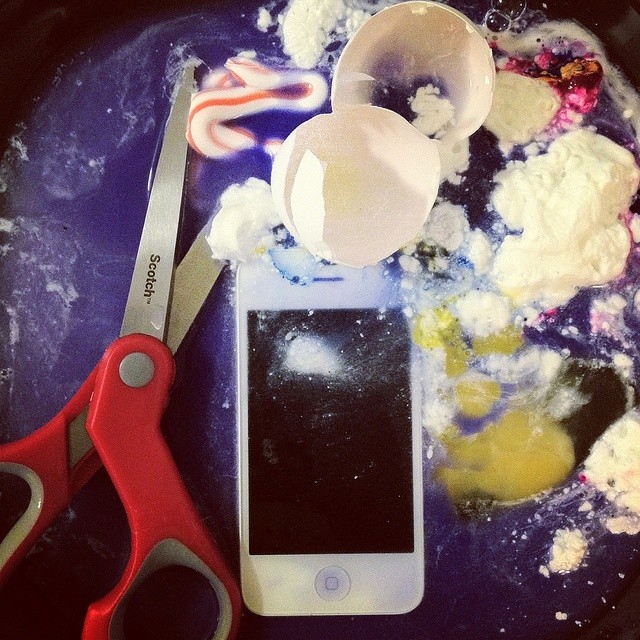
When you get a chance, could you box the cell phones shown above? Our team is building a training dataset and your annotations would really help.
[236,255,426,618]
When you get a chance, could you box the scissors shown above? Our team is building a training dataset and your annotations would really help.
[0,63,241,640]
